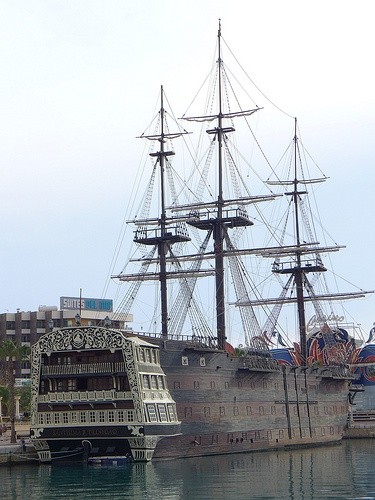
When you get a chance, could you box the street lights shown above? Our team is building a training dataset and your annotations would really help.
[2,338,21,443]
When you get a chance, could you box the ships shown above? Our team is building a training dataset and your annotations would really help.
[231,117,375,438]
[27,19,347,460]
